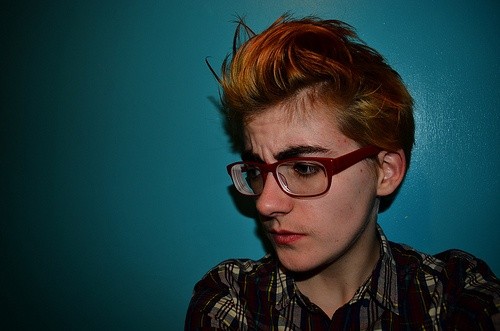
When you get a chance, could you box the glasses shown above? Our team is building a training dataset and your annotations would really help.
[228,144,377,197]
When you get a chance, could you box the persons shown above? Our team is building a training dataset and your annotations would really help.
[182,10,500,331]
